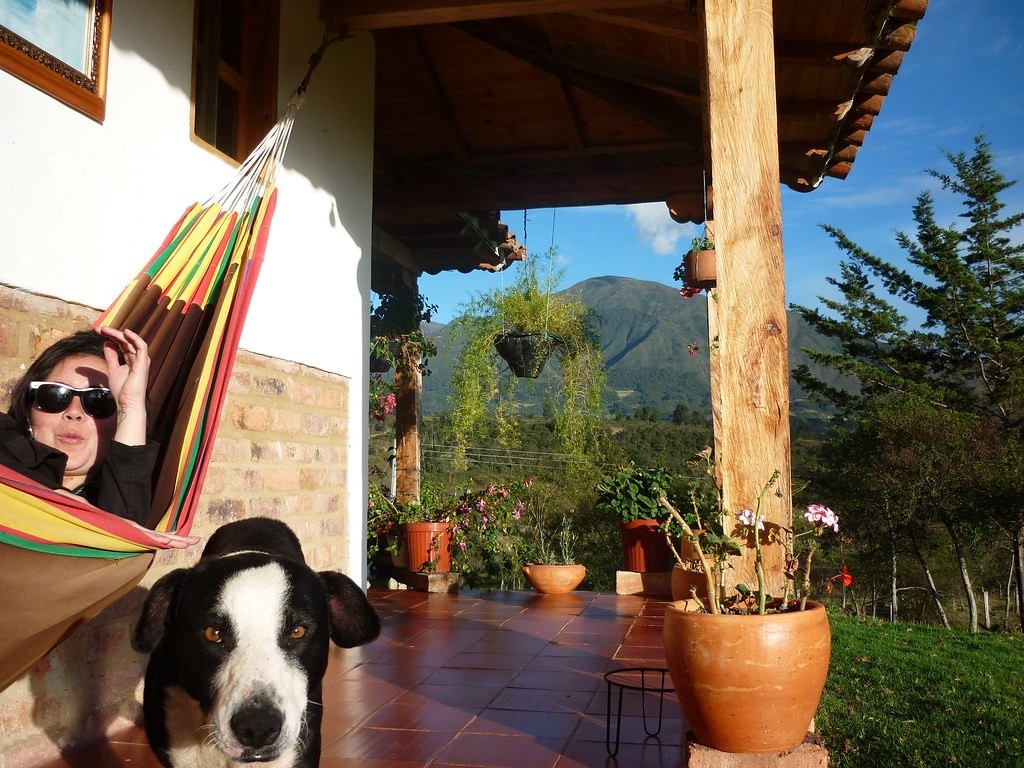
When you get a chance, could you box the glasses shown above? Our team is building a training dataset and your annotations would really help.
[28,380,117,420]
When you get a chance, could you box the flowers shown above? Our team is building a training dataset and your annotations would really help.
[683,446,722,480]
[723,473,867,596]
[677,281,706,302]
[366,373,402,424]
[441,473,541,556]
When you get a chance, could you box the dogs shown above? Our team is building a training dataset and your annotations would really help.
[129,514,383,768]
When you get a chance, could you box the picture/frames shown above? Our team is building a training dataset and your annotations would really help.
[0,0,115,123]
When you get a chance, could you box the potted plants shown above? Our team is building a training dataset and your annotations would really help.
[599,458,682,573]
[665,483,717,602]
[360,275,440,373]
[670,238,718,288]
[445,247,607,488]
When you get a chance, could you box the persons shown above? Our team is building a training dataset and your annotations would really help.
[0,326,160,524]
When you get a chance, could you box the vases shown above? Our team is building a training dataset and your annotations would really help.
[384,522,457,575]
[681,527,717,561]
[521,561,586,592]
[664,593,832,756]
